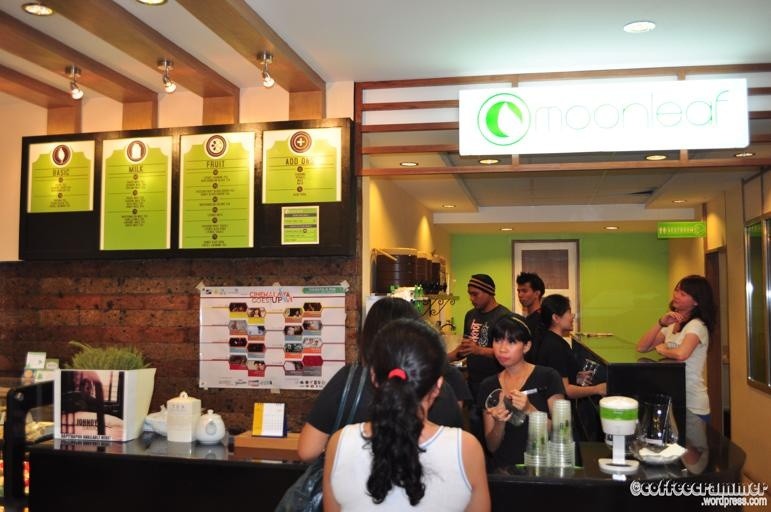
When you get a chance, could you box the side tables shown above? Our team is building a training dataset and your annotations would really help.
[29,332,747,512]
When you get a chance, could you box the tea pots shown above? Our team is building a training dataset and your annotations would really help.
[196,410,225,446]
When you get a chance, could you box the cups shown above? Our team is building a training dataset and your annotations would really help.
[524,411,549,467]
[548,400,575,467]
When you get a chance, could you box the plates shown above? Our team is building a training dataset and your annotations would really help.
[630,443,679,465]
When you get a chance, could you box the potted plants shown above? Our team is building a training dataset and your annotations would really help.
[54,340,157,441]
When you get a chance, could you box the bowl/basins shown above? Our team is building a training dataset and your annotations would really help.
[146,412,168,437]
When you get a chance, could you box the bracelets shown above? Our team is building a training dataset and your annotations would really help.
[658,317,669,328]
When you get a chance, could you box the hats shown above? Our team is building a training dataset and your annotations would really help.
[468,272,496,298]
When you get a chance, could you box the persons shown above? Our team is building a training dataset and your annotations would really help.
[447,272,516,385]
[444,360,472,411]
[297,296,466,467]
[470,314,567,467]
[322,318,492,512]
[635,274,715,425]
[63,379,96,412]
[517,272,546,327]
[536,293,612,399]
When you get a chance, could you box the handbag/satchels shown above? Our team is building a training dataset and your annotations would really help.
[275,360,367,512]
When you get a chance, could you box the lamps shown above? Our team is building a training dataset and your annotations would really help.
[258,54,275,89]
[65,67,84,100]
[156,58,177,94]
